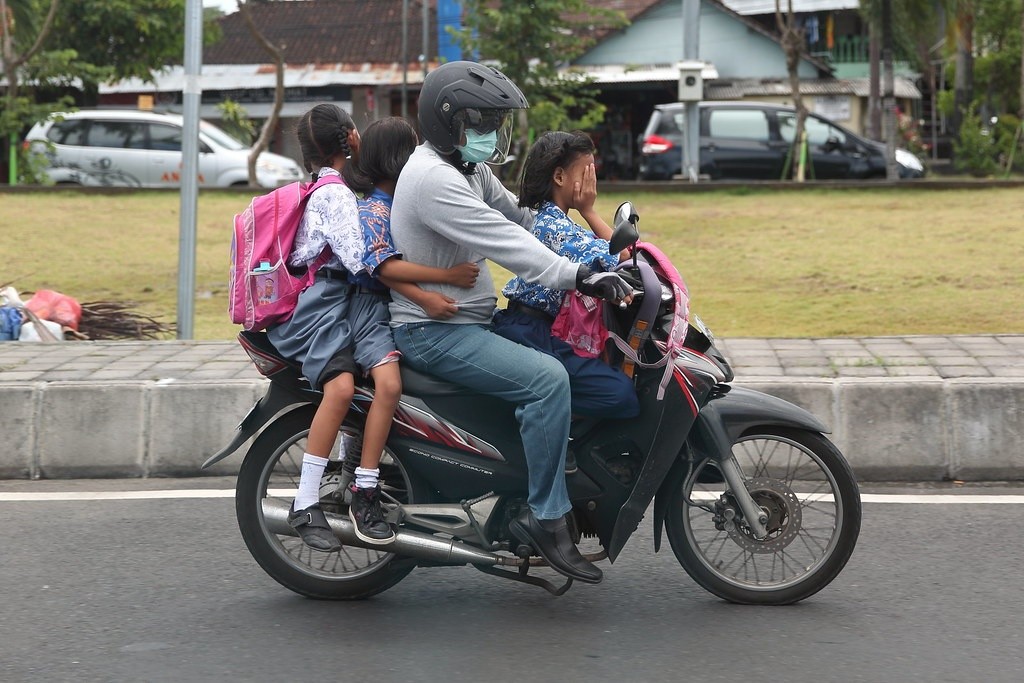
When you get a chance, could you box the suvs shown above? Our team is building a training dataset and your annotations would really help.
[20,110,305,192]
[639,100,924,181]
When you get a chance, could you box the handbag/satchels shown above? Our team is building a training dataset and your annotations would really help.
[23,289,82,330]
[18,319,63,342]
[0,287,24,307]
[0,307,23,340]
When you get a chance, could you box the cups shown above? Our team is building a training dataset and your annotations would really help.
[253,258,278,304]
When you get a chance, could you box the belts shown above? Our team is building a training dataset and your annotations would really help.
[290,267,346,278]
[359,287,381,295]
[507,301,554,322]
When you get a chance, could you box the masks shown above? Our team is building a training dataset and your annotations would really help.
[453,128,499,164]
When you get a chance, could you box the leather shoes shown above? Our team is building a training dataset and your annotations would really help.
[508,509,603,584]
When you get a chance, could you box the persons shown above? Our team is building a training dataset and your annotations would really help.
[264,103,460,552]
[387,60,634,583]
[494,131,640,474]
[341,118,479,543]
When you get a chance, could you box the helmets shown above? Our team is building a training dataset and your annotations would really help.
[417,60,530,165]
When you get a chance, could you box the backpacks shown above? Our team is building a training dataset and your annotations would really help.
[228,175,348,333]
[550,234,689,399]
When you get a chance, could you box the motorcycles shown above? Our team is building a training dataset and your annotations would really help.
[202,201,862,606]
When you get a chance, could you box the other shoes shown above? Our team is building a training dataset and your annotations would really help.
[565,449,577,473]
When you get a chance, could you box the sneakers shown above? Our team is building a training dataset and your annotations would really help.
[286,499,343,552]
[348,485,395,545]
